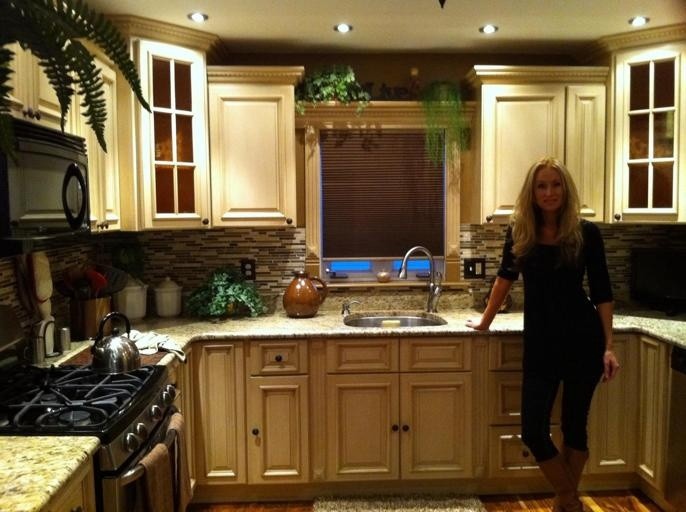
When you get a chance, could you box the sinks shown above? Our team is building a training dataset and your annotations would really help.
[344,310,448,328]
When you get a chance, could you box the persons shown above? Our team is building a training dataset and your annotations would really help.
[466,155,620,512]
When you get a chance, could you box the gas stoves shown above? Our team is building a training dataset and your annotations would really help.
[1,361,170,440]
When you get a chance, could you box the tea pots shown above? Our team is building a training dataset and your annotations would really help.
[90,312,141,374]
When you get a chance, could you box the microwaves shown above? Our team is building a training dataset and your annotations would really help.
[2,113,95,240]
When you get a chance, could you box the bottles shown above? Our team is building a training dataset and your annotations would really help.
[112,272,149,323]
[153,276,184,319]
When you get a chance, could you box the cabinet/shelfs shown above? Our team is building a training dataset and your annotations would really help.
[462,82,607,227]
[118,36,208,233]
[606,38,686,227]
[194,341,310,503]
[169,349,195,511]
[1,39,116,234]
[484,337,639,494]
[206,82,297,229]
[324,336,474,496]
[638,336,685,507]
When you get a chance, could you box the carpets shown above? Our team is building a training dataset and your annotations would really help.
[313,495,487,512]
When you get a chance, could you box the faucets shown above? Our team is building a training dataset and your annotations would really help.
[399,246,435,284]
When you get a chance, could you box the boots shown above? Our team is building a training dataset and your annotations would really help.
[535,445,591,511]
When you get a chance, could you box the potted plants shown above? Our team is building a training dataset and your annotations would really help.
[182,267,268,320]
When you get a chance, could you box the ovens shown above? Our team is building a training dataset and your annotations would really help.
[99,370,189,512]
